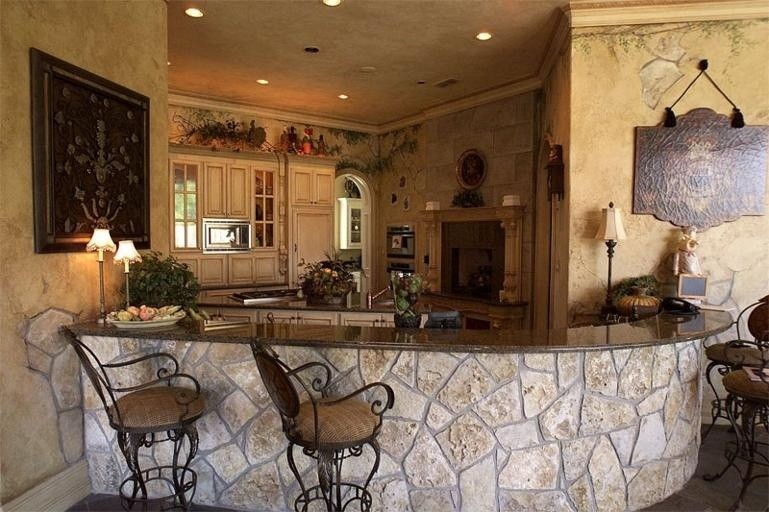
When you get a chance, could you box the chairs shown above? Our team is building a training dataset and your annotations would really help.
[249,342,394,512]
[60,325,205,511]
[703,294,768,438]
[704,319,769,511]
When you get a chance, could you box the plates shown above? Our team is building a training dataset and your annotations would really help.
[108,315,184,330]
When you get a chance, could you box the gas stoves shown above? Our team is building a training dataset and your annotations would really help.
[227,289,304,305]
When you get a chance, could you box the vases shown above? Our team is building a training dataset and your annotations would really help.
[391,271,425,330]
[307,297,347,306]
[615,285,661,315]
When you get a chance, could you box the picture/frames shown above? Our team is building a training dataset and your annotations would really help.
[30,47,150,254]
[455,147,490,192]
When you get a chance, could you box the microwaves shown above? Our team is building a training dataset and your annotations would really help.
[201,217,253,255]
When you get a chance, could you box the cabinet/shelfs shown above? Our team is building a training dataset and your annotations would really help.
[289,205,335,291]
[202,161,251,218]
[259,309,337,338]
[202,306,256,336]
[339,312,395,341]
[291,167,334,204]
[173,254,225,285]
[229,254,280,283]
[337,198,362,250]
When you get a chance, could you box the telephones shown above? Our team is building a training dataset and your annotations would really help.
[661,295,700,314]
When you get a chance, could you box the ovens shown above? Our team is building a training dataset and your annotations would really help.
[385,226,418,285]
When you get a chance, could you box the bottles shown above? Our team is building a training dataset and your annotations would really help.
[365,289,373,308]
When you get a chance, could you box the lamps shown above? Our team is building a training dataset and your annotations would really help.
[86,229,116,323]
[595,202,626,315]
[114,241,142,308]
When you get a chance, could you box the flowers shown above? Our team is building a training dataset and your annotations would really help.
[300,261,357,296]
